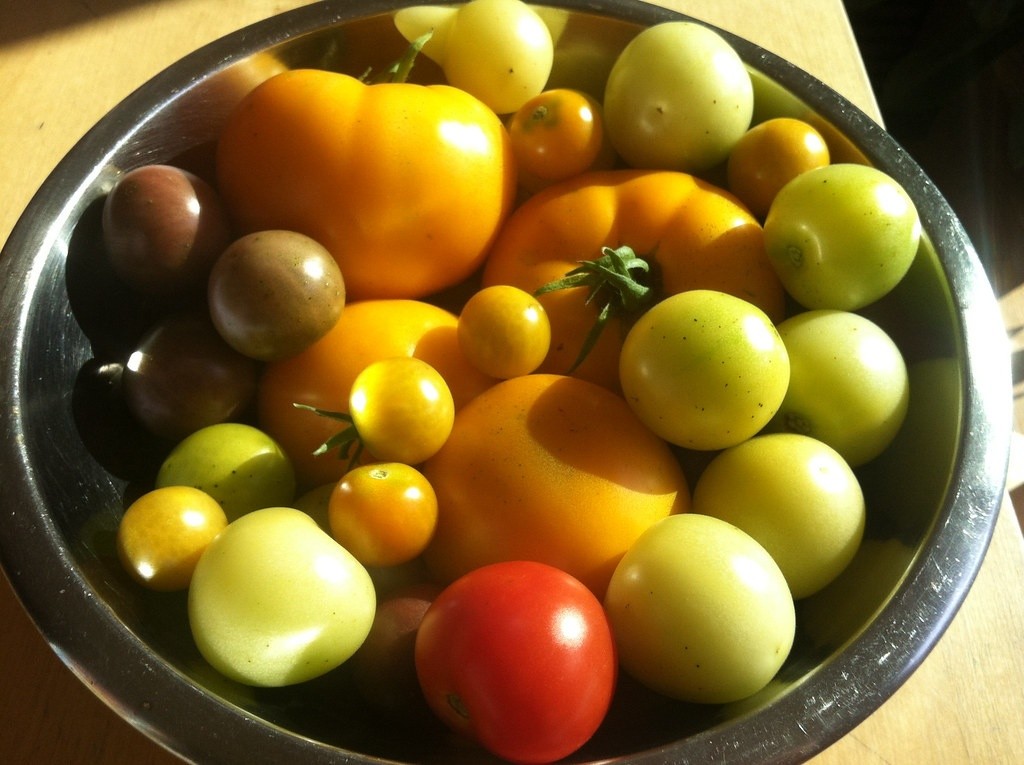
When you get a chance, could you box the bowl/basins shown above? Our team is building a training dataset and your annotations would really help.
[0,0,1024,765]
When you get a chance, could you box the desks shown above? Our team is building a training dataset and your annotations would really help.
[0,0,1024,765]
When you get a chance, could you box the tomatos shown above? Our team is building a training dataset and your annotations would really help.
[91,1,918,765]
